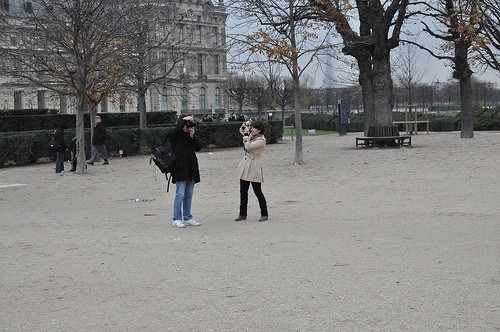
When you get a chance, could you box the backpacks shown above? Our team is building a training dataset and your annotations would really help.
[149,128,174,173]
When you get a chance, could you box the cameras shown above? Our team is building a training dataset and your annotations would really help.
[248,123,252,129]
[187,121,199,128]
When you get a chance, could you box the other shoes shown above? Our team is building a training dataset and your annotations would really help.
[103,161,108,165]
[235,216,246,221]
[87,162,94,165]
[259,216,268,221]
[173,219,185,228]
[183,219,200,226]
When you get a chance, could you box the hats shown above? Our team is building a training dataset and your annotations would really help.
[96,116,101,119]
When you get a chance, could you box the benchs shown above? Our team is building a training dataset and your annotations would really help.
[356,135,411,149]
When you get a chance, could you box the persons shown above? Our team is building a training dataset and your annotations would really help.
[235,121,268,221]
[51,126,67,174]
[86,115,108,165]
[170,115,201,227]
[228,113,245,121]
[69,129,87,172]
[202,114,218,122]
